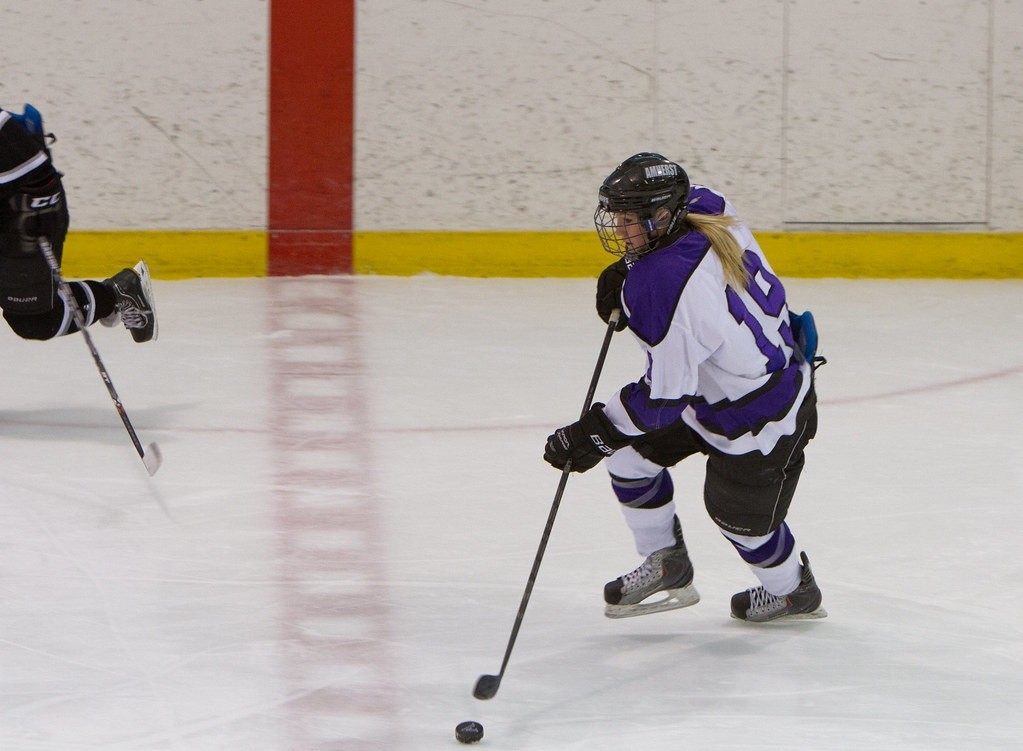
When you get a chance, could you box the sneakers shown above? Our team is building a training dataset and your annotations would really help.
[603,515,699,619]
[103,259,159,343]
[730,552,828,624]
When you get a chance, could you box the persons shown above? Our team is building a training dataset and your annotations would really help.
[0,104,158,344]
[544,151,830,621]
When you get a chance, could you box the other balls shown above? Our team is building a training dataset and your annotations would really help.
[455,721,484,745]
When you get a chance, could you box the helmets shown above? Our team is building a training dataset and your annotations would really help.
[594,152,693,261]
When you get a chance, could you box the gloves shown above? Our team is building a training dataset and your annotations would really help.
[543,403,637,473]
[10,182,62,257]
[596,258,629,332]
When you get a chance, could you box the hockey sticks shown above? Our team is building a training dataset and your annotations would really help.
[38,235,164,476]
[472,307,621,701]
